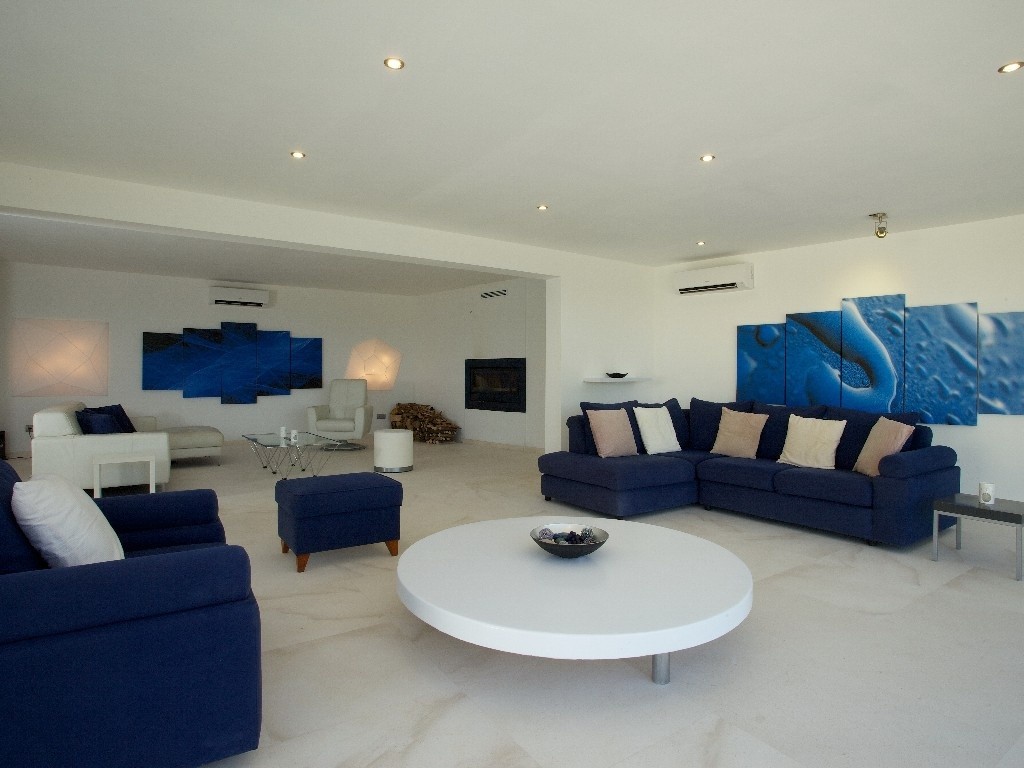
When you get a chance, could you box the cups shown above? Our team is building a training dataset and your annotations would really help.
[280,427,287,437]
[290,429,298,441]
[978,482,994,505]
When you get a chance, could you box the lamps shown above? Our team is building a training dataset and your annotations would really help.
[11,317,110,396]
[341,340,403,392]
[868,212,888,238]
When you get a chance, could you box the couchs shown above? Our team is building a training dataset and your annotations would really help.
[0,460,262,768]
[30,402,225,491]
[539,399,960,548]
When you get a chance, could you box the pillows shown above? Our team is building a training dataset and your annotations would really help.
[586,408,639,458]
[11,470,126,568]
[775,414,847,470]
[632,406,682,455]
[710,407,770,459]
[75,404,138,434]
[851,416,915,477]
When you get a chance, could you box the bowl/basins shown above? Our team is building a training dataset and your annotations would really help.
[530,523,609,559]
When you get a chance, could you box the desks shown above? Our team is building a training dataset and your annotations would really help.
[933,492,1024,582]
[373,429,414,473]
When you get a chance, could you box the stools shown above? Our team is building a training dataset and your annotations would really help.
[275,471,404,572]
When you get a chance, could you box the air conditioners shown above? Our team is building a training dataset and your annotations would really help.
[208,287,271,309]
[673,263,754,296]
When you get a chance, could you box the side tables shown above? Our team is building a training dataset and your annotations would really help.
[92,453,155,498]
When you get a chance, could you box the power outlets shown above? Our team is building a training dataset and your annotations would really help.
[25,425,33,432]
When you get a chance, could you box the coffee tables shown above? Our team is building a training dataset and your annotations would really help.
[395,516,753,684]
[241,432,343,481]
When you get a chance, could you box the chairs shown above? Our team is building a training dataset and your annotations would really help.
[306,379,374,451]
